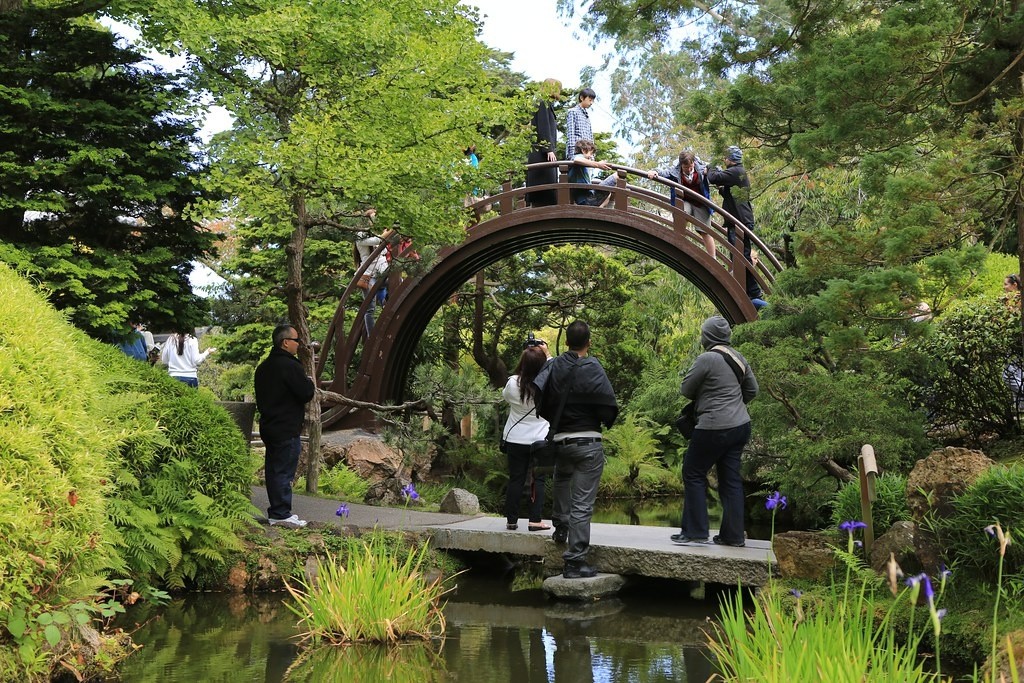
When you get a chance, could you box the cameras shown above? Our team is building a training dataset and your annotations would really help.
[524,333,543,350]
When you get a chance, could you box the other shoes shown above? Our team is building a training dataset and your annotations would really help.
[507,523,518,529]
[268,515,307,528]
[528,521,551,530]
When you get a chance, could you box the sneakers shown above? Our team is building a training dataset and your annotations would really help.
[713,534,745,546]
[671,534,709,542]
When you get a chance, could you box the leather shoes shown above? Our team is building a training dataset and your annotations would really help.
[553,530,567,541]
[563,561,598,577]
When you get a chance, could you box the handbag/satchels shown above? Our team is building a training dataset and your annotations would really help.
[357,275,370,290]
[465,193,492,213]
[500,432,507,453]
[387,238,420,261]
[675,401,697,438]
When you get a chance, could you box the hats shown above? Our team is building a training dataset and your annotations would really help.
[138,315,143,322]
[724,146,743,163]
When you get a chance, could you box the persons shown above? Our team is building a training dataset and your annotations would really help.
[162,321,215,389]
[893,291,930,341]
[526,79,562,206]
[502,341,553,531]
[746,249,768,309]
[254,325,315,526]
[671,318,759,548]
[647,151,722,260]
[534,320,618,578]
[354,209,419,338]
[112,315,147,361]
[568,140,617,207]
[708,146,754,274]
[1003,274,1024,409]
[566,88,597,161]
[437,143,478,229]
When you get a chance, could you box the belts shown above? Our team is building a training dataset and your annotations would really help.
[556,436,601,446]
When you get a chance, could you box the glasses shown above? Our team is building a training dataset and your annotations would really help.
[1010,274,1017,281]
[281,337,300,343]
